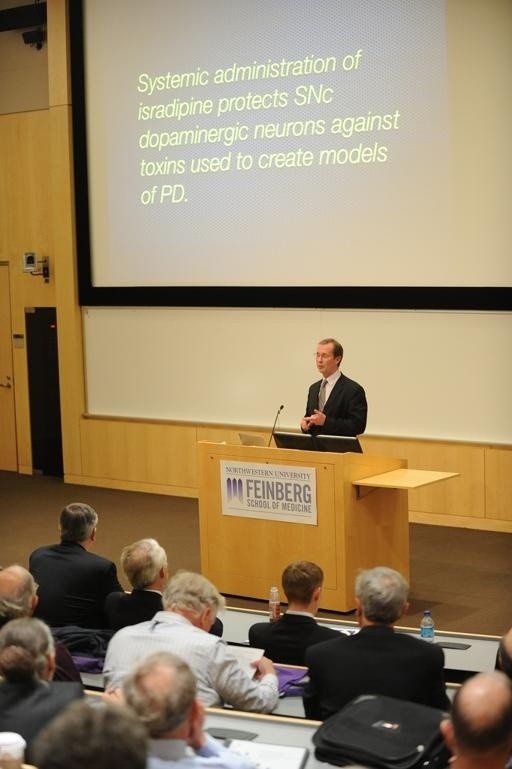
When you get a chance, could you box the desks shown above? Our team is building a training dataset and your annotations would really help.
[79,689,362,769]
[216,605,502,679]
[71,647,460,721]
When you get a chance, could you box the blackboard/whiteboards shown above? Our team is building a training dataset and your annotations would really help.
[82,305,512,449]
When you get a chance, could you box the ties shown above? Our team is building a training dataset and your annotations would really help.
[317,380,328,413]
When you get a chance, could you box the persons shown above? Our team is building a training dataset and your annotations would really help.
[125,649,261,769]
[101,570,280,713]
[497,628,512,673]
[29,501,124,627]
[104,538,223,640]
[248,560,348,667]
[440,670,511,768]
[303,565,456,723]
[25,689,148,769]
[302,337,368,436]
[1,564,85,763]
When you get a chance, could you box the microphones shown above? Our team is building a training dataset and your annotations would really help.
[267,405,285,444]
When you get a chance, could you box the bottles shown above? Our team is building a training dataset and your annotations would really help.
[420,611,435,643]
[270,587,280,622]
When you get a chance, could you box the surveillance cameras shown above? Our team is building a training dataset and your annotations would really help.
[22,250,48,273]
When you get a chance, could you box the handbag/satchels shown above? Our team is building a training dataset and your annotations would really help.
[312,693,453,768]
